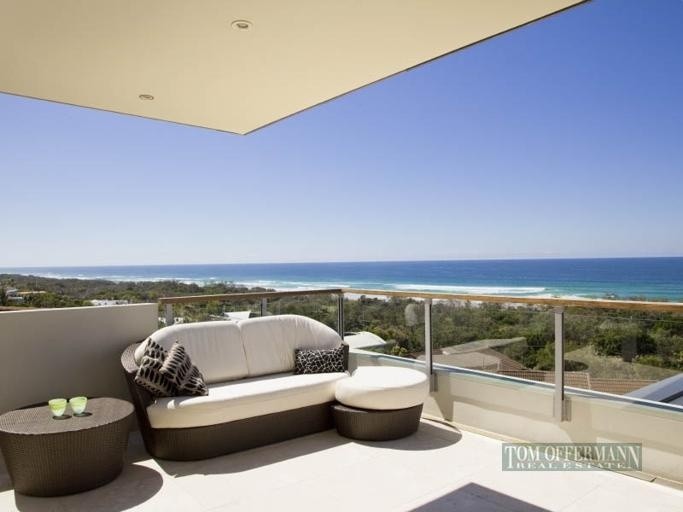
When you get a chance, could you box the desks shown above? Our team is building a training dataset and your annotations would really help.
[0,397,135,497]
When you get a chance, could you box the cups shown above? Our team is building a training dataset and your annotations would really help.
[47,397,67,417]
[68,396,87,415]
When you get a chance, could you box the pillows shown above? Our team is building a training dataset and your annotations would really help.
[159,340,212,396]
[134,337,177,397]
[293,344,347,375]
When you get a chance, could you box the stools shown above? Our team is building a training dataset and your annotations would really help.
[331,367,427,441]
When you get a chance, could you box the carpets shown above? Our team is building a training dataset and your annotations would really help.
[407,482,553,512]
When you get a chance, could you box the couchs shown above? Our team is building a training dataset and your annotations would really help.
[119,314,349,460]
